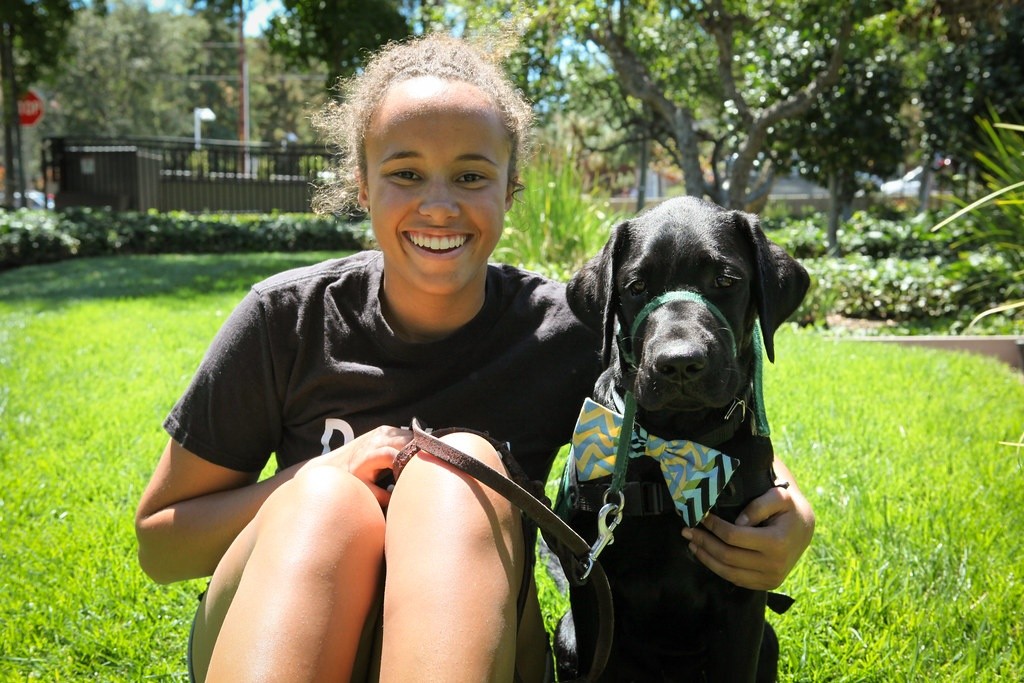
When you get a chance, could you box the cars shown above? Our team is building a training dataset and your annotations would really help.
[879,157,962,199]
[0,188,56,214]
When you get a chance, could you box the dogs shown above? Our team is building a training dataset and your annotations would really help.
[553,194,811,683]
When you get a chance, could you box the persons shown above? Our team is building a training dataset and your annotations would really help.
[134,34,815,683]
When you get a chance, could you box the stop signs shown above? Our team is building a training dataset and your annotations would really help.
[16,90,42,128]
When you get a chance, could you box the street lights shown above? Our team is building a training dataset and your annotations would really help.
[194,107,217,176]
